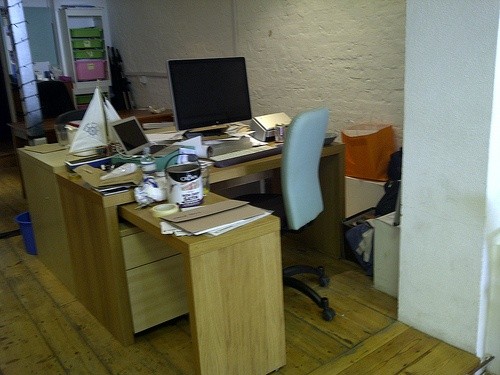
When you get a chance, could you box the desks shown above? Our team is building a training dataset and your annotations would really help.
[15,111,401,375]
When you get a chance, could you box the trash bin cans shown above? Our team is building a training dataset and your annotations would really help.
[16,210,38,255]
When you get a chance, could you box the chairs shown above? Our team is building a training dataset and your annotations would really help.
[231,107,338,322]
[38,81,76,120]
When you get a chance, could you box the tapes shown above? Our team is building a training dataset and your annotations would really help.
[152,204,179,218]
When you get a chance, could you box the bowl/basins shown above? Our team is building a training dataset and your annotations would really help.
[323,132,337,146]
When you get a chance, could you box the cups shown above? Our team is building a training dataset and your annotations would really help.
[54,124,67,145]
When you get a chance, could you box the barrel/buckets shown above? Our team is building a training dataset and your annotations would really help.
[163,154,204,207]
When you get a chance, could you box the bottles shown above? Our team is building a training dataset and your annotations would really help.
[141,148,157,180]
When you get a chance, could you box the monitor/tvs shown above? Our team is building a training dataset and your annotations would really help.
[168,56,251,138]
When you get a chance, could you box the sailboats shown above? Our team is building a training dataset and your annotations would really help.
[65,78,127,173]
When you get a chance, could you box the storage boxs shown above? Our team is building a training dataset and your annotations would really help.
[70,28,107,110]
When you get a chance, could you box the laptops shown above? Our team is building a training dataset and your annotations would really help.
[111,115,181,157]
